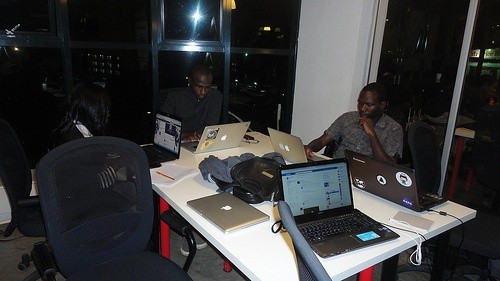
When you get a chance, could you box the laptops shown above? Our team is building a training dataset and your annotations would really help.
[181,121,251,154]
[268,126,328,164]
[344,148,450,212]
[278,157,400,259]
[187,191,271,234]
[141,114,183,164]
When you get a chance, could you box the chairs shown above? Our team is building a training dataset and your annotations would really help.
[23,138,194,280]
[0,118,49,238]
[408,122,448,199]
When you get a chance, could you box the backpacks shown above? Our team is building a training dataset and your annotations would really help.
[231,156,285,203]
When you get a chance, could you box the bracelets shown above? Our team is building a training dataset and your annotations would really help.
[370,135,377,142]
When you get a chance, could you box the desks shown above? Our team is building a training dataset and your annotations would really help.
[142,132,477,281]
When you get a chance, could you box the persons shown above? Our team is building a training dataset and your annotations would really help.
[54,82,109,142]
[163,64,222,257]
[475,75,497,107]
[303,82,404,163]
